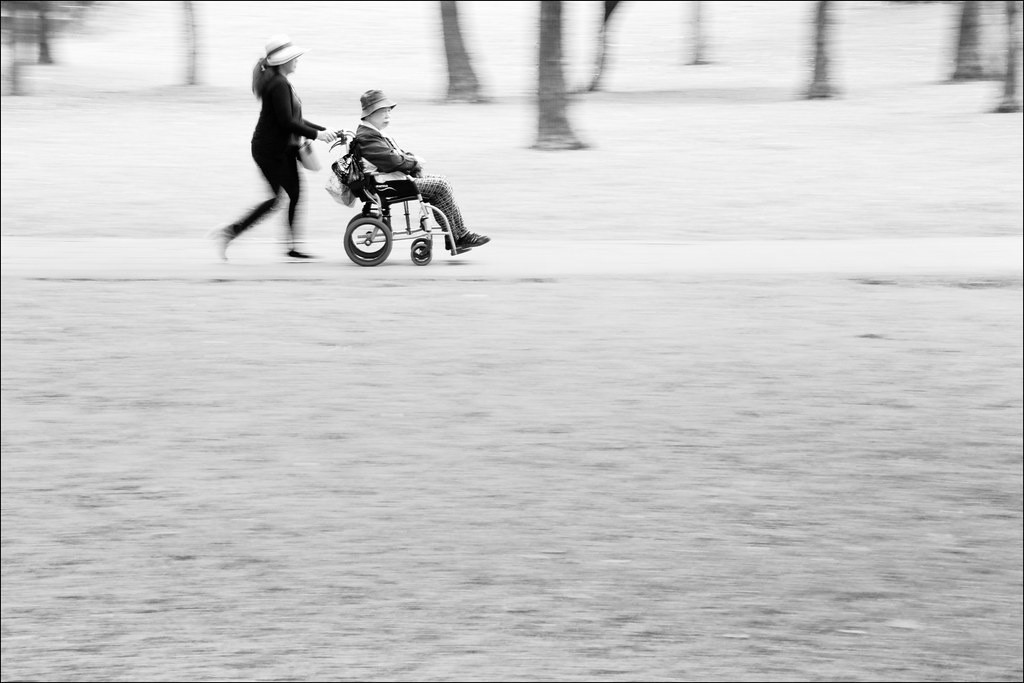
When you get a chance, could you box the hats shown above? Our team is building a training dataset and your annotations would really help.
[265,36,310,66]
[360,89,397,120]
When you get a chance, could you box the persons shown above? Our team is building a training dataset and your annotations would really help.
[211,33,338,262]
[353,89,491,250]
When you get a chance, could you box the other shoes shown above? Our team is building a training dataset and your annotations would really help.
[444,233,491,251]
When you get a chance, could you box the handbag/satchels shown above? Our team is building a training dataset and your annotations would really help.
[299,141,322,171]
[331,154,367,198]
[325,170,356,208]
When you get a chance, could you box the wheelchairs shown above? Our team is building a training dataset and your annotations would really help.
[324,129,473,267]
